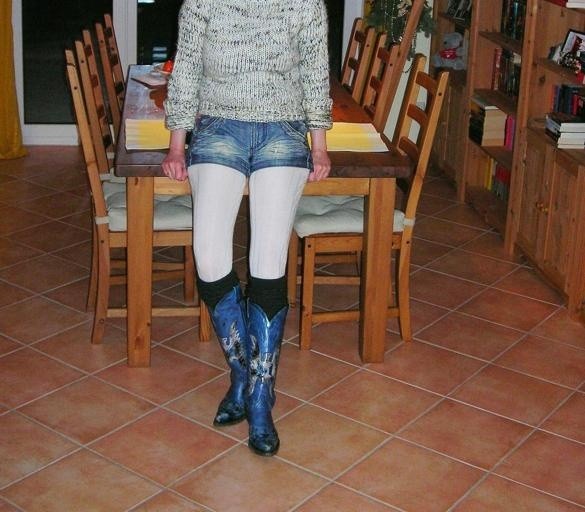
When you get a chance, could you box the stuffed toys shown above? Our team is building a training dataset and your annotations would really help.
[432,31,468,71]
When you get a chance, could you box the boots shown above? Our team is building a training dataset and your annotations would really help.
[244,298,288,457]
[207,286,246,427]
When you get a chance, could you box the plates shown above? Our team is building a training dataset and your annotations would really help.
[153,62,174,75]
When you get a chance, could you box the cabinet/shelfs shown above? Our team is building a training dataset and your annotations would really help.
[361,1,585,327]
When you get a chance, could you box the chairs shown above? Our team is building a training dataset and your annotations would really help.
[358,34,401,131]
[342,22,376,101]
[96,13,126,136]
[66,44,211,344]
[74,27,117,173]
[289,55,450,351]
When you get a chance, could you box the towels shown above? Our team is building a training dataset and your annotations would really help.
[308,121,388,152]
[125,118,170,151]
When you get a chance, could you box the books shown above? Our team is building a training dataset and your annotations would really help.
[500,0,527,43]
[547,0,585,9]
[491,46,522,102]
[483,156,511,204]
[468,96,516,153]
[543,82,585,150]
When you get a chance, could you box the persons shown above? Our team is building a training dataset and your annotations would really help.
[161,0,334,458]
[455,0,471,19]
